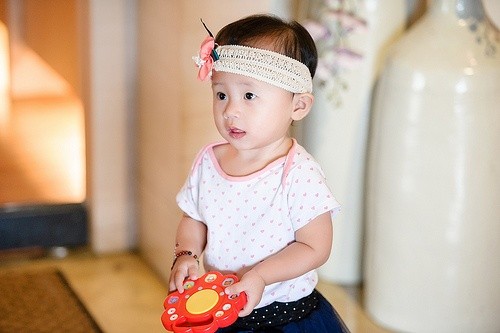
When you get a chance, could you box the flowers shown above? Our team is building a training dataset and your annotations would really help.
[192,38,217,80]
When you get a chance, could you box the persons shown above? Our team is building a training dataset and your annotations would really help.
[169,17,350,333]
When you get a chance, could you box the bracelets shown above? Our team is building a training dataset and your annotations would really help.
[170,249,199,268]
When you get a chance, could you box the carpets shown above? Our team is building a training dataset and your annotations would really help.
[0,269,103,333]
